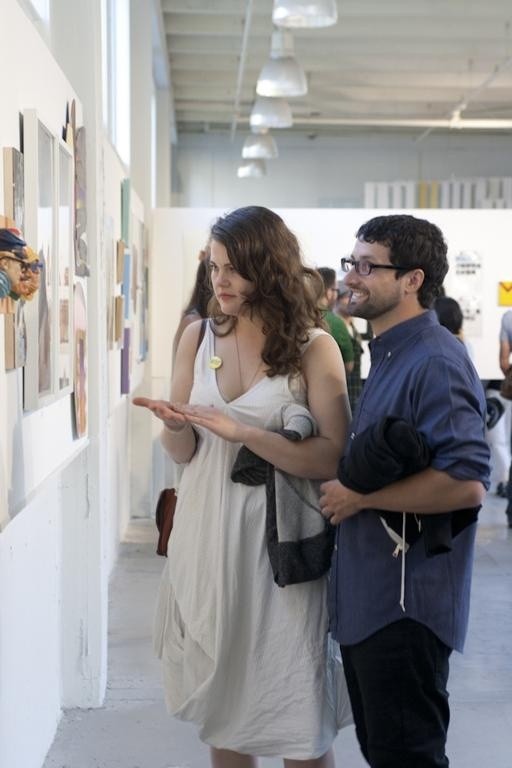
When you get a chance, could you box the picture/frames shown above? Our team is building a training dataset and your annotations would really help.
[20,107,77,413]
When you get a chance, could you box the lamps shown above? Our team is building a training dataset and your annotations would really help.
[234,2,340,179]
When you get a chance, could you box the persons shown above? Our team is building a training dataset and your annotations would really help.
[303,267,363,403]
[132,206,352,768]
[435,295,462,339]
[0,228,41,301]
[319,215,491,767]
[173,259,213,358]
[497,311,512,497]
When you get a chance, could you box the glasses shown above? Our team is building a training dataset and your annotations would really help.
[341,257,409,277]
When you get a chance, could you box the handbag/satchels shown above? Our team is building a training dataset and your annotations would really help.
[156,488,177,557]
